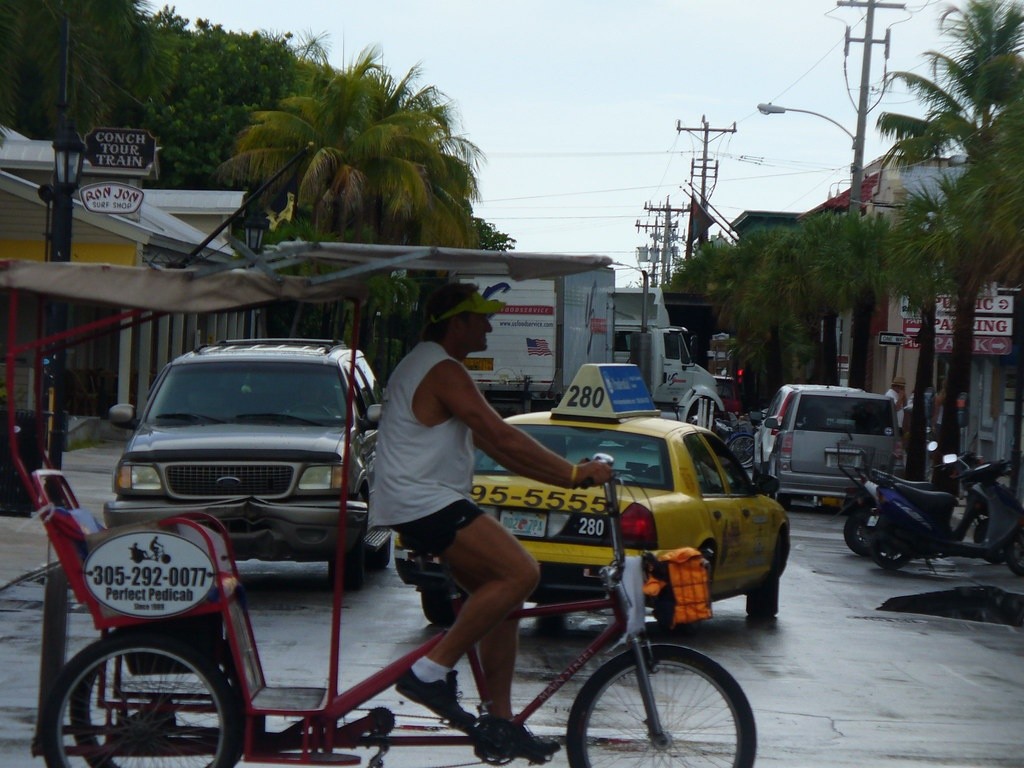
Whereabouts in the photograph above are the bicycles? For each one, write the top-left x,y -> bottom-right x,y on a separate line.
721,410 -> 773,471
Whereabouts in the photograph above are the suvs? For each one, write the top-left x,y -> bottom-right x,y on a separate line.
103,337 -> 393,594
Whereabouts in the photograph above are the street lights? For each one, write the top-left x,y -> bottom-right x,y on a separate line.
757,100 -> 866,391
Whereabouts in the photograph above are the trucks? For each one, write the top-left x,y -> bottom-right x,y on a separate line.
446,253 -> 719,434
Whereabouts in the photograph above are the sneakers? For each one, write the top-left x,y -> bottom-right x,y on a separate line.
476,715 -> 561,756
395,668 -> 477,728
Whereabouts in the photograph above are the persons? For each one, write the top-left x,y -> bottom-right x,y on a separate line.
366,282 -> 614,754
931,377 -> 948,438
884,376 -> 907,433
287,377 -> 338,416
715,366 -> 722,375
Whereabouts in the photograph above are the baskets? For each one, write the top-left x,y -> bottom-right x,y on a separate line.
645,555 -> 714,628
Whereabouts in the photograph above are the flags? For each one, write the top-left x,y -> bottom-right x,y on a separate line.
686,197 -> 716,259
233,168 -> 299,233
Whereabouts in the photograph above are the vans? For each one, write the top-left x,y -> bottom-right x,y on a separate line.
751,384 -> 906,516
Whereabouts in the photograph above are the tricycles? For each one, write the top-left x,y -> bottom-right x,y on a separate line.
0,243 -> 756,768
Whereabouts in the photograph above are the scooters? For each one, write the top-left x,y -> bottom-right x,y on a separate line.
838,444 -> 1024,579
831,432 -> 1008,573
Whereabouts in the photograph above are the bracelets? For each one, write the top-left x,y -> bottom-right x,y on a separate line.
570,464 -> 578,489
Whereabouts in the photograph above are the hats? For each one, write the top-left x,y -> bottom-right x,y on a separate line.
430,291 -> 507,324
892,377 -> 907,387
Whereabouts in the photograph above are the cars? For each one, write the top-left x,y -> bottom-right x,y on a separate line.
713,374 -> 742,421
390,362 -> 790,640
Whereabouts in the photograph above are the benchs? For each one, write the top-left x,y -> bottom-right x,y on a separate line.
34,469 -> 243,630
